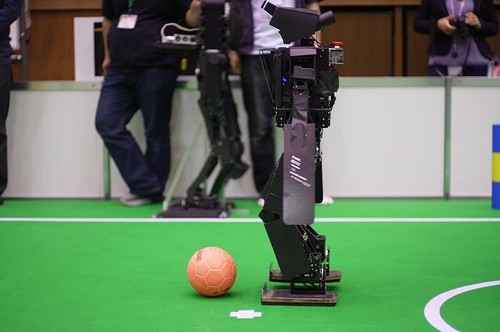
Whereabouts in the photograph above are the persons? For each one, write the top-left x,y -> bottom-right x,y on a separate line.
257,0 -> 343,308
94,0 -> 201,206
412,0 -> 500,76
0,0 -> 22,206
224,0 -> 334,207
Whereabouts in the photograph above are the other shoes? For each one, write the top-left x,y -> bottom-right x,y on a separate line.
121,195 -> 150,206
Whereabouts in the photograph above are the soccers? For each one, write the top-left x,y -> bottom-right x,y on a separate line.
186,247 -> 237,297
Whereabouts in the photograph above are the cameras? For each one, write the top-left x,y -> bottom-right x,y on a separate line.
449,16 -> 470,38
